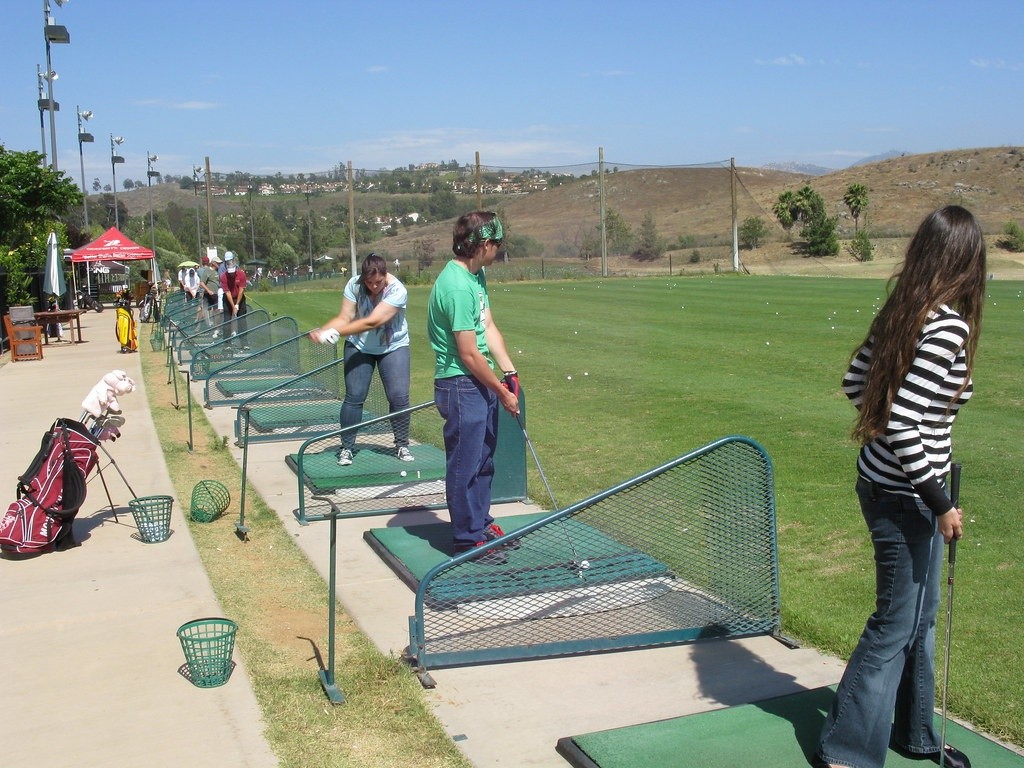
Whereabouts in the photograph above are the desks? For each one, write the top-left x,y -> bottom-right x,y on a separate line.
33,309 -> 87,347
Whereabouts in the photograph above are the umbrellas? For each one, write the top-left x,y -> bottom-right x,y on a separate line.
43,229 -> 67,340
177,260 -> 200,268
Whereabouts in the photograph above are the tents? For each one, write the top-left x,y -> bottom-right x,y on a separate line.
72,226 -> 159,310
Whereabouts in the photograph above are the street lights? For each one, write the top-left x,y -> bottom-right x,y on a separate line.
146,155 -> 160,253
38,69 -> 60,169
44,0 -> 72,173
304,187 -> 312,267
78,109 -> 95,238
110,136 -> 124,231
194,166 -> 203,265
247,177 -> 257,271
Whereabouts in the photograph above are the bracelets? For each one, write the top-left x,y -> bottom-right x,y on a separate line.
503,371 -> 517,376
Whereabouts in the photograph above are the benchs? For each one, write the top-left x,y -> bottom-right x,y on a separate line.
4,314 -> 44,361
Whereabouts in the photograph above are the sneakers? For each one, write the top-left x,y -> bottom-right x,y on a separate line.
483,523 -> 522,550
335,448 -> 353,466
454,538 -> 510,566
393,446 -> 414,462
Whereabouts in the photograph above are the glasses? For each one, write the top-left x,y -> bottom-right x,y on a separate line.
490,239 -> 502,246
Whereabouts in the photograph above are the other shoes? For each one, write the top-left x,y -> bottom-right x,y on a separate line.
811,752 -> 832,768
212,330 -> 224,339
928,742 -> 972,768
244,345 -> 250,350
224,346 -> 231,351
231,331 -> 237,338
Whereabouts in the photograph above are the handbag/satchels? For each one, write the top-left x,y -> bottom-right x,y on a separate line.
115,305 -> 139,352
138,295 -> 153,322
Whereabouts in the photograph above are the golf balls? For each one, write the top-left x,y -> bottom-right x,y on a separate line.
581,560 -> 589,568
401,471 -> 407,477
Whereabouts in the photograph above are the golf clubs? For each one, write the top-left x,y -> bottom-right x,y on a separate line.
245,294 -> 278,316
938,461 -> 972,768
164,313 -> 211,359
232,314 -> 237,337
89,405 -> 126,443
505,374 -> 580,567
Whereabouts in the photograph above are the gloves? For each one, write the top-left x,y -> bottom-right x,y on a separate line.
500,370 -> 519,401
235,304 -> 240,311
318,328 -> 340,346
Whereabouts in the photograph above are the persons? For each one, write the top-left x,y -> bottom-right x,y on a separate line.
308,253 -> 415,465
178,252 -> 251,351
426,211 -> 523,566
162,267 -> 172,294
812,205 -> 986,768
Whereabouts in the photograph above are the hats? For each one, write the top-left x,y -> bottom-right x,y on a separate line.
149,282 -> 155,287
224,251 -> 234,261
189,269 -> 194,277
202,256 -> 209,262
211,256 -> 223,264
227,260 -> 237,273
